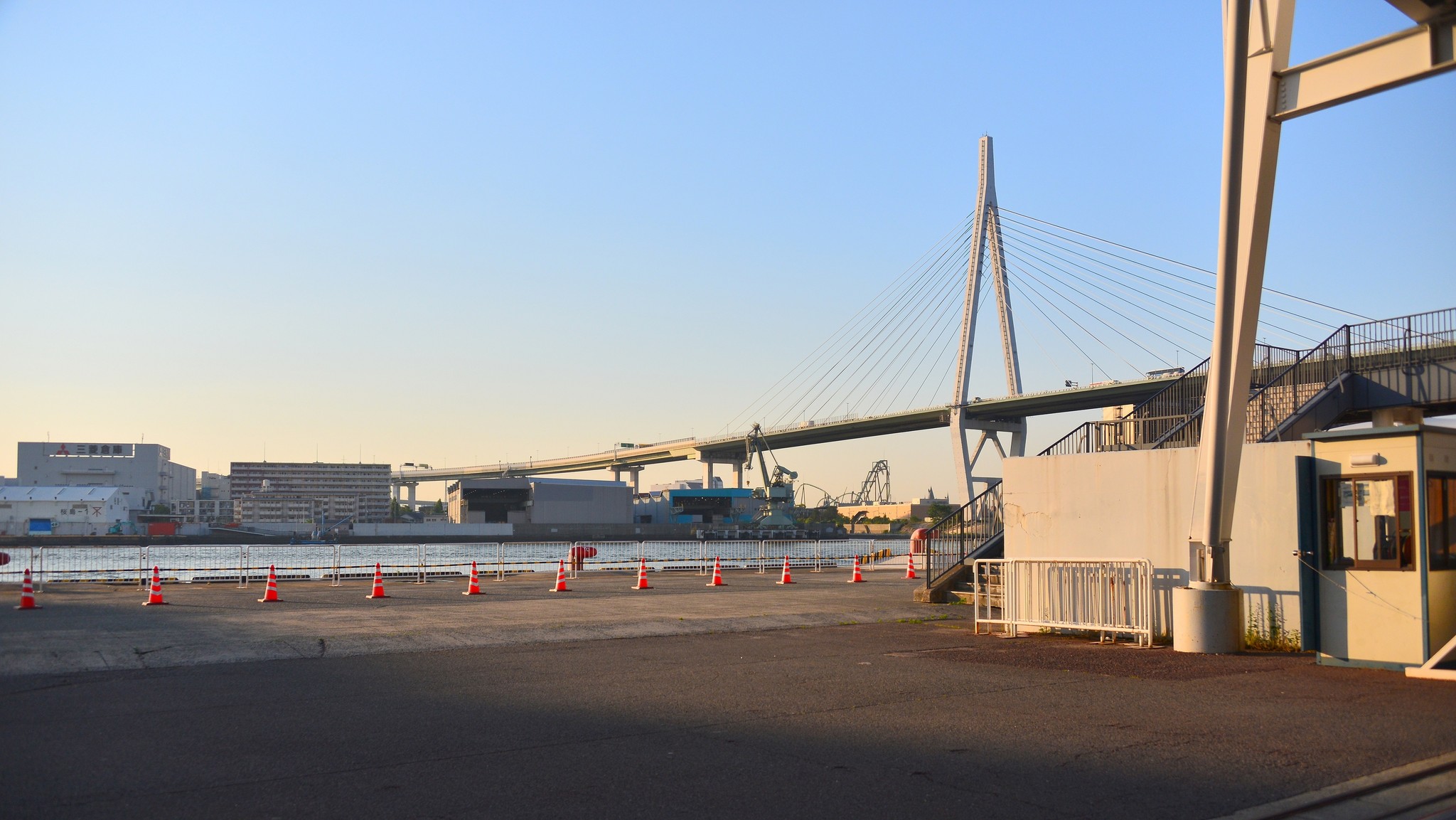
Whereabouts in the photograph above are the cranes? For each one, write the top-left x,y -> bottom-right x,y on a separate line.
743,423 -> 799,528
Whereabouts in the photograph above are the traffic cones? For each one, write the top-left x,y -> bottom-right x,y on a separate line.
706,556 -> 728,586
257,565 -> 283,602
365,563 -> 391,599
14,569 -> 43,610
776,555 -> 797,585
142,565 -> 170,606
462,561 -> 487,596
847,555 -> 867,583
901,553 -> 921,579
630,558 -> 654,589
549,559 -> 573,592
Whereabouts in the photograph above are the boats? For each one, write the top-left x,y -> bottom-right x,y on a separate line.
289,509 -> 354,544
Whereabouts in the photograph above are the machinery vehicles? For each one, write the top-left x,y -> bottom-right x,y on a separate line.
105,521 -> 138,535
